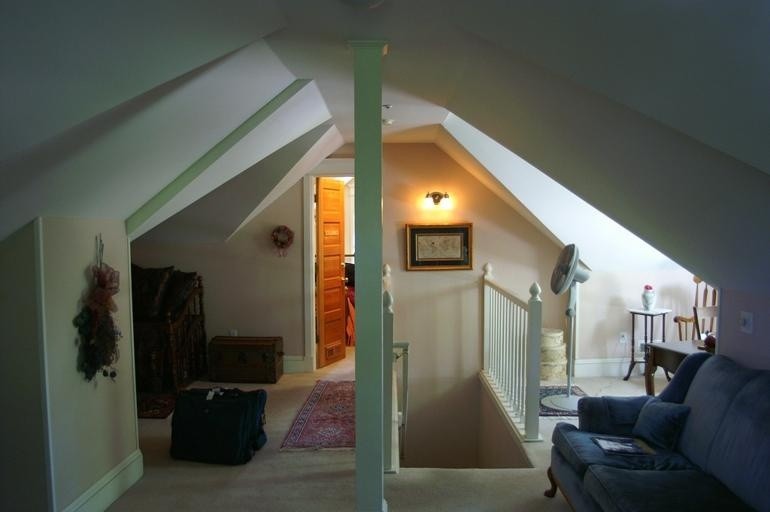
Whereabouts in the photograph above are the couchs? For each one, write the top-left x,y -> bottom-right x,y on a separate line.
543,352 -> 770,510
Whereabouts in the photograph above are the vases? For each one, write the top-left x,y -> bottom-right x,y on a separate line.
641,290 -> 657,312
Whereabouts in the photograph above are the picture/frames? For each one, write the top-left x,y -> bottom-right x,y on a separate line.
405,223 -> 474,271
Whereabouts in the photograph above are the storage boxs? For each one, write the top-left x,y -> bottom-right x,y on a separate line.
208,335 -> 284,384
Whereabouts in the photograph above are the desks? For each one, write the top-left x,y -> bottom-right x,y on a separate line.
644,339 -> 709,397
623,308 -> 672,382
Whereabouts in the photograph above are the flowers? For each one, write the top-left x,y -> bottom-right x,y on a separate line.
644,285 -> 653,290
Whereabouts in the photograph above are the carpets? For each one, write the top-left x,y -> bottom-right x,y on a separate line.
539,384 -> 590,417
279,379 -> 355,452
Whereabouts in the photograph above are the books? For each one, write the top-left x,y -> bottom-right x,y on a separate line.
591,435 -> 657,457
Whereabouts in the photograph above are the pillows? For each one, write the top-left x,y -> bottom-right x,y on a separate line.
632,397 -> 691,452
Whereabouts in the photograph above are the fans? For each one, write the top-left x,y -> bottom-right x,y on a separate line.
540,244 -> 593,412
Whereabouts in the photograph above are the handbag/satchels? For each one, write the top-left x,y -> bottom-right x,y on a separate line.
171,387 -> 267,465
